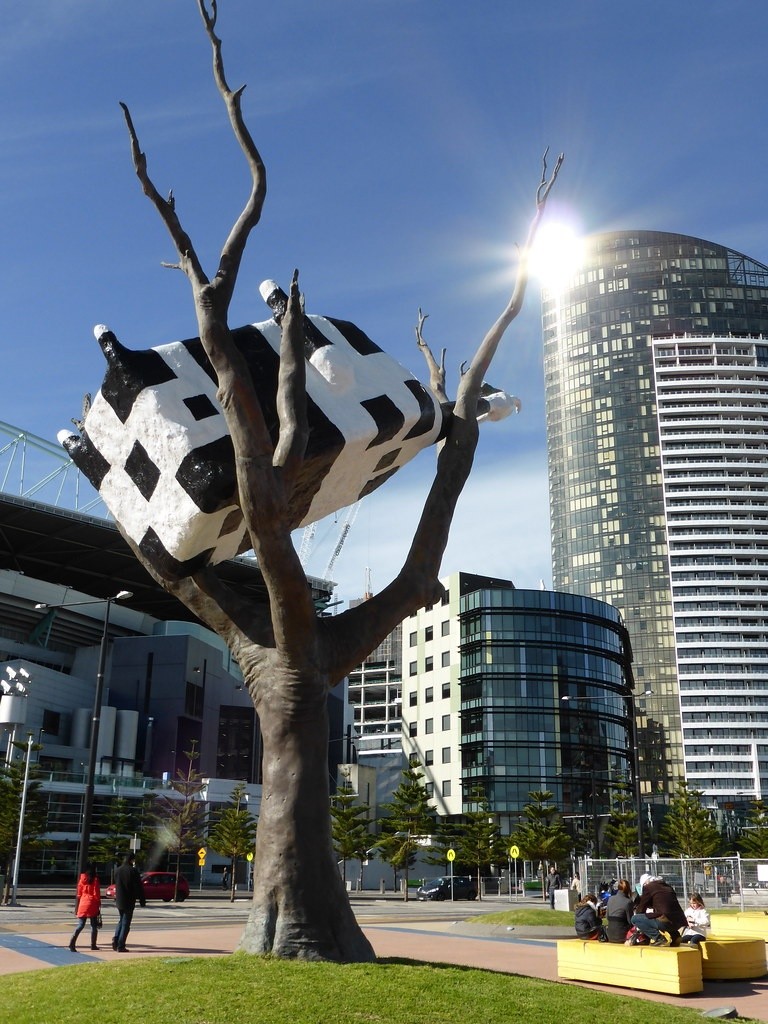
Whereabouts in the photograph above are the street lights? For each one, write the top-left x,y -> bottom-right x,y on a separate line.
34,590 -> 134,914
555,769 -> 616,903
561,691 -> 654,877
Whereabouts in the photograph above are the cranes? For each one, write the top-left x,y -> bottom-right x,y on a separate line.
299,498 -> 363,580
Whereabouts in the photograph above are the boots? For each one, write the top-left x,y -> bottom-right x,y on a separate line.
68,930 -> 81,952
91,931 -> 99,950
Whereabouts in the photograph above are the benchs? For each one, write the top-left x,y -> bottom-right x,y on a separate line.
557,913 -> 768,995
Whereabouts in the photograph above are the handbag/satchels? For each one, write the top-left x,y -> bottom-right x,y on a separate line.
628,930 -> 650,945
95,911 -> 102,929
597,925 -> 609,942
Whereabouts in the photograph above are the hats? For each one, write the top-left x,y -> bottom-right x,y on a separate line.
640,874 -> 651,886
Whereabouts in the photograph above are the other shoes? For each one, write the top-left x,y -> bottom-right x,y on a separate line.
112,937 -> 119,950
650,935 -> 668,946
117,947 -> 129,952
670,936 -> 682,947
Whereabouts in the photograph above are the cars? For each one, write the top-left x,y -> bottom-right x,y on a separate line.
105,871 -> 189,903
416,875 -> 478,902
661,876 -> 689,897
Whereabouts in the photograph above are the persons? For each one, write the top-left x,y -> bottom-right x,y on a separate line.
545,867 -> 562,909
112,852 -> 145,952
69,860 -> 101,952
250,867 -> 253,891
570,863 -> 733,947
222,867 -> 228,891
